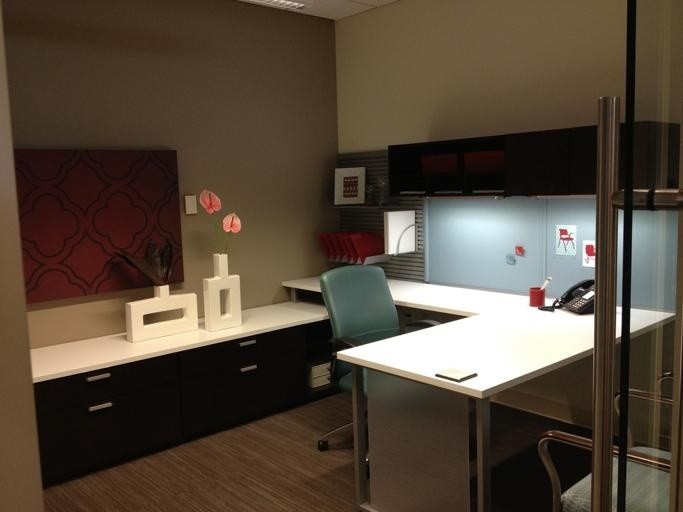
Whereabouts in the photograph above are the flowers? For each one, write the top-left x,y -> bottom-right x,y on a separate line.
197,188 -> 246,256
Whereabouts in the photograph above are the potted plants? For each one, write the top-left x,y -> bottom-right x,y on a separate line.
109,239 -> 198,344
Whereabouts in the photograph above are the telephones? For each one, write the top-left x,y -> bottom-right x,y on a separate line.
559,280 -> 596,313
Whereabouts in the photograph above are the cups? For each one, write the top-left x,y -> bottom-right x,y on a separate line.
529,287 -> 546,308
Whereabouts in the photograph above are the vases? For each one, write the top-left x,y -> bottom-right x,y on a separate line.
203,252 -> 242,331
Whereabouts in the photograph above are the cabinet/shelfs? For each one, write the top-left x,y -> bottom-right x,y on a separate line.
32,355 -> 183,489
178,332 -> 278,447
268,318 -> 335,417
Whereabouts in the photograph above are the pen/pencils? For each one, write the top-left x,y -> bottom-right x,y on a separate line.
540,277 -> 552,290
